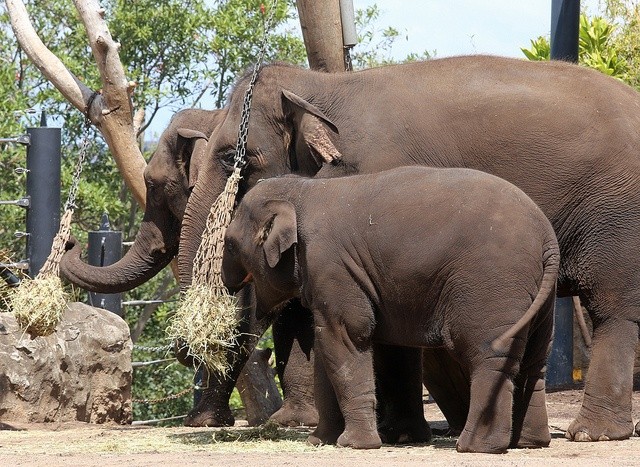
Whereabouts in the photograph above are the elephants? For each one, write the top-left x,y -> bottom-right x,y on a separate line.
56,105 -> 471,437
179,53 -> 640,443
220,163 -> 562,455
